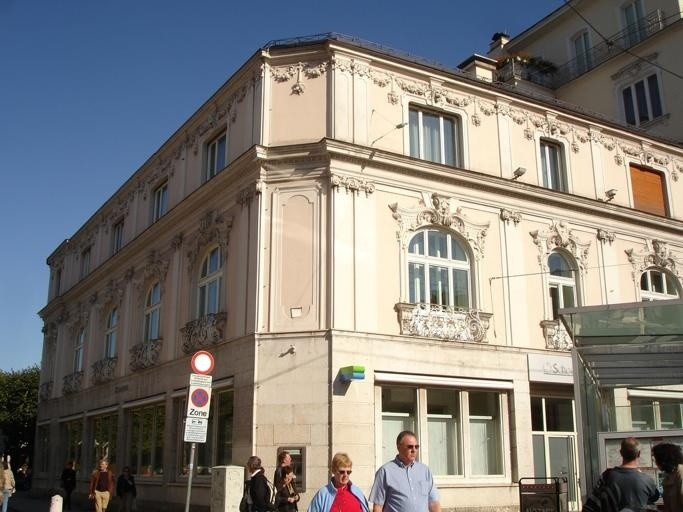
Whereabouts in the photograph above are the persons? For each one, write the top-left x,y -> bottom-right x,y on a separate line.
0,461 -> 32,512
368,430 -> 442,512
117,466 -> 137,512
592,437 -> 660,512
650,440 -> 683,512
273,449 -> 296,488
306,453 -> 370,512
252,467 -> 269,512
89,459 -> 116,512
60,461 -> 77,510
244,455 -> 275,512
276,466 -> 301,512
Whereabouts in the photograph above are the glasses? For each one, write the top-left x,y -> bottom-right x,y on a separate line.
335,468 -> 353,475
400,444 -> 420,449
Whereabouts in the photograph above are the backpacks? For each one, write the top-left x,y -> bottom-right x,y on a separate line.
265,479 -> 281,512
581,469 -> 626,512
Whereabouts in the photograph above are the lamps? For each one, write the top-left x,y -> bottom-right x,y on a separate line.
372,122 -> 408,145
604,188 -> 619,203
511,167 -> 526,180
